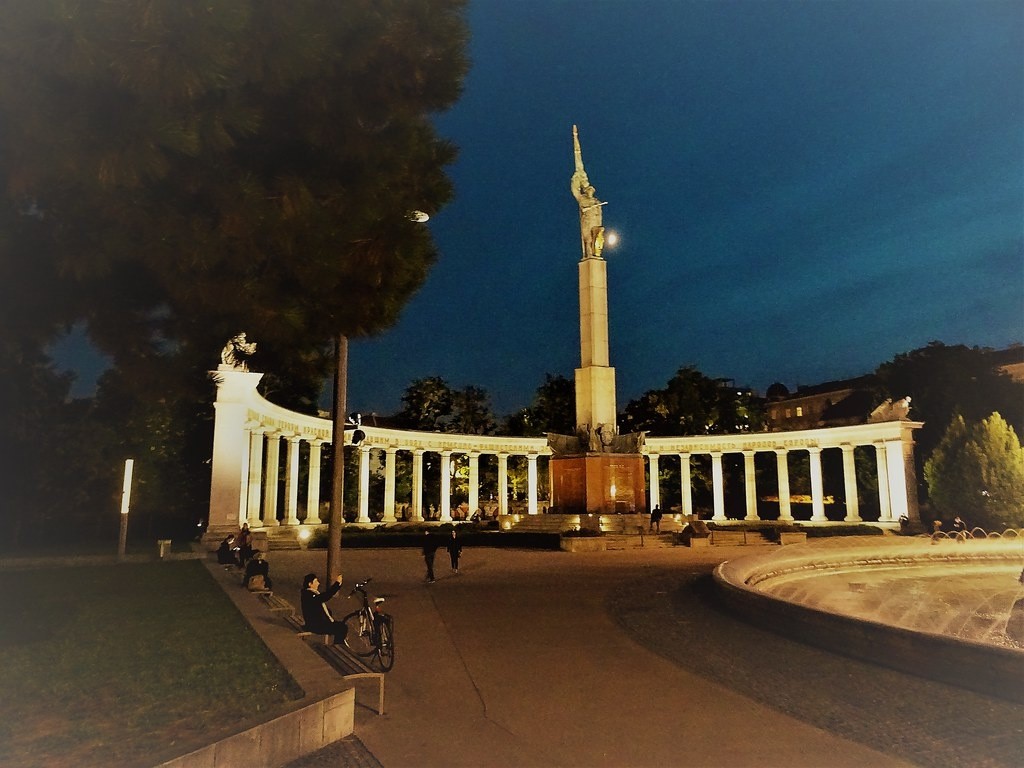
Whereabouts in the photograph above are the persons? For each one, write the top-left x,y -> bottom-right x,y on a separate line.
577,186 -> 603,259
447,529 -> 462,573
241,553 -> 274,591
216,535 -> 241,563
649,503 -> 662,534
239,523 -> 249,547
301,572 -> 348,644
954,517 -> 966,540
422,529 -> 436,584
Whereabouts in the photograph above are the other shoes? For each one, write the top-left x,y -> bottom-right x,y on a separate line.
452,569 -> 458,573
428,579 -> 435,583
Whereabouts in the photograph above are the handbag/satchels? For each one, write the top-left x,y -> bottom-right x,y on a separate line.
248,575 -> 265,591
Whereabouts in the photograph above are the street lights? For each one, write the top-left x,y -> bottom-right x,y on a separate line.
328,207 -> 430,598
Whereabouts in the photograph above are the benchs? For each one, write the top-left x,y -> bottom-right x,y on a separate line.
259,595 -> 296,616
223,564 -> 273,596
310,644 -> 384,716
282,614 -> 329,645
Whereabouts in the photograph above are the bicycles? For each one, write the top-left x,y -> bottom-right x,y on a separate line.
341,577 -> 395,674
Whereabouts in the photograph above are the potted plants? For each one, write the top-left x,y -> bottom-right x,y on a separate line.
560,527 -> 607,553
775,524 -> 807,546
